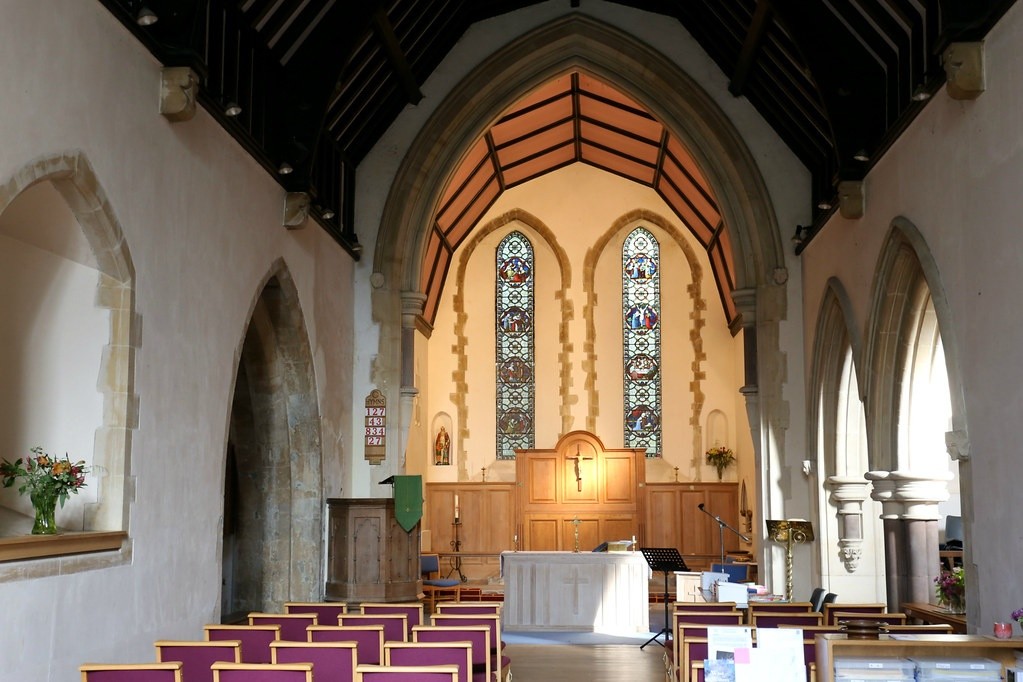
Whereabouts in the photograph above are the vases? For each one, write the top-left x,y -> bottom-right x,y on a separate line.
717,463 -> 723,482
944,595 -> 966,614
30,493 -> 58,534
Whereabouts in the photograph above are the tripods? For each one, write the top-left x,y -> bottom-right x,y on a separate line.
639,547 -> 692,649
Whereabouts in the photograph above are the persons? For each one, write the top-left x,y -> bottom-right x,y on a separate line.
435,427 -> 450,465
567,453 -> 592,479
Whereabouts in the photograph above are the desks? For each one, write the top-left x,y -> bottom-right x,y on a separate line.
815,634 -> 1023,682
499,550 -> 652,633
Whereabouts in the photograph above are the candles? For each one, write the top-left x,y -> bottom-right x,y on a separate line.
454,495 -> 458,518
632,535 -> 635,542
514,535 -> 517,542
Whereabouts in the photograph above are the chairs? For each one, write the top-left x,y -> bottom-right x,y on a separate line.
663,588 -> 954,682
78,601 -> 511,682
421,554 -> 460,616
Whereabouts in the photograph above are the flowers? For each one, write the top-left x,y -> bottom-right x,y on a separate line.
0,448 -> 91,527
1009,608 -> 1023,623
933,569 -> 966,610
706,447 -> 736,478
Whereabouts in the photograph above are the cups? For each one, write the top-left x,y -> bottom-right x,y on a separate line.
994,623 -> 1012,639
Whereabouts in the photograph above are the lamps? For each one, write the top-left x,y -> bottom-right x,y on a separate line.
771,69 -> 935,244
136,5 -> 364,250
